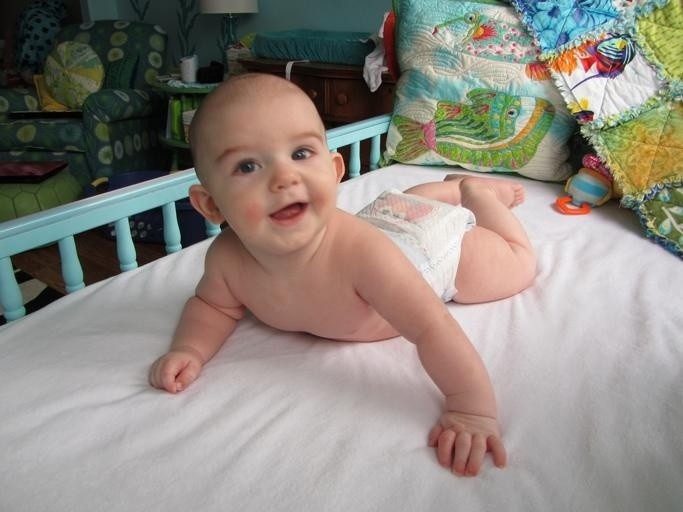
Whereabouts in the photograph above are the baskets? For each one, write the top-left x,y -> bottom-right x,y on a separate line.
85,169 -> 204,245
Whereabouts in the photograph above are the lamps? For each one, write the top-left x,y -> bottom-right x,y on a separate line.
198,0 -> 259,45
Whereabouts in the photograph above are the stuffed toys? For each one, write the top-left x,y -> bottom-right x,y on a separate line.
556,154 -> 620,216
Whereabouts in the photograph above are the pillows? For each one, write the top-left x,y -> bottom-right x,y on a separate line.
374,0 -> 578,186
34,37 -> 142,114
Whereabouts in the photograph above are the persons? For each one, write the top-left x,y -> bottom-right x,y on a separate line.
148,74 -> 540,480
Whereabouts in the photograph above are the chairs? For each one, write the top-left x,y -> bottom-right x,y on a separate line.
0,18 -> 169,189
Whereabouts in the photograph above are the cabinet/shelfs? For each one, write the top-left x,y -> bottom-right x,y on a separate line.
236,56 -> 396,184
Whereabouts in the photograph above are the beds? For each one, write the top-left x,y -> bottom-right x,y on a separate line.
1,113 -> 683,512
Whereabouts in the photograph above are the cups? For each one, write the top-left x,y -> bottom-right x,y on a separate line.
179,54 -> 199,84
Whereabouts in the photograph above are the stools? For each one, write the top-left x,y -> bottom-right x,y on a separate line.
0,171 -> 82,250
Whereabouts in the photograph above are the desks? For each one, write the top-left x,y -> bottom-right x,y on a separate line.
151,83 -> 221,172
